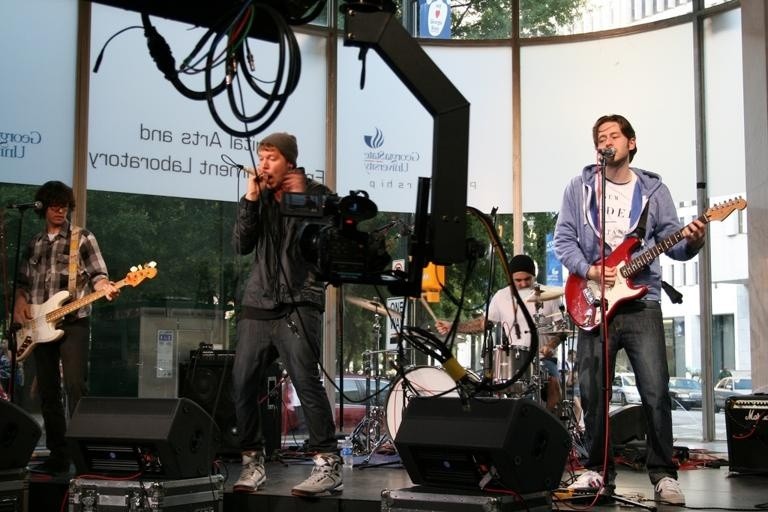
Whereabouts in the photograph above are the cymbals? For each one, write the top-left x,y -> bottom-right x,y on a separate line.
521,287 -> 564,302
540,326 -> 580,337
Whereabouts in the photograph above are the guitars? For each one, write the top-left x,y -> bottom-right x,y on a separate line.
7,260 -> 158,362
564,196 -> 746,331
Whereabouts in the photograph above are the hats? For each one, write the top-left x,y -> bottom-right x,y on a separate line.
259,131 -> 298,168
507,254 -> 536,277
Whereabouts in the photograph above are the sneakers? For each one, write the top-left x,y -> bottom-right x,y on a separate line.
653,476 -> 686,505
567,469 -> 616,492
290,451 -> 345,497
232,450 -> 267,493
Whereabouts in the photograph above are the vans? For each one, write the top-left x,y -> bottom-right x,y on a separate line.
281,377 -> 391,434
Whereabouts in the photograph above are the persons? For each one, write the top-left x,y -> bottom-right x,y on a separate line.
0,337 -> 14,403
553,115 -> 705,506
232,132 -> 345,498
11,180 -> 121,476
436,255 -> 569,414
559,349 -> 576,400
718,367 -> 732,388
567,361 -> 582,425
29,358 -> 64,400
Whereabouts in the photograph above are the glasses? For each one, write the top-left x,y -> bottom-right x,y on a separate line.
47,203 -> 73,213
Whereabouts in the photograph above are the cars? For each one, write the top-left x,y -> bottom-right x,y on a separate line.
713,377 -> 756,411
608,371 -> 643,406
666,377 -> 703,409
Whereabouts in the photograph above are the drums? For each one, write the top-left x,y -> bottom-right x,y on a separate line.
387,364 -> 465,443
482,344 -> 540,394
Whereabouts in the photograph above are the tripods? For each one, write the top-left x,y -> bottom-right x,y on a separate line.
347,319 -> 586,469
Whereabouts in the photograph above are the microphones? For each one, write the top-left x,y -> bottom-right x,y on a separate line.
598,147 -> 616,159
241,166 -> 269,182
6,201 -> 43,210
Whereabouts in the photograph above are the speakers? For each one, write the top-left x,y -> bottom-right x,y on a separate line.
608,403 -> 647,444
189,349 -> 281,460
725,395 -> 768,478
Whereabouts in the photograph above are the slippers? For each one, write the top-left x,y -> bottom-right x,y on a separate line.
25,462 -> 70,475
